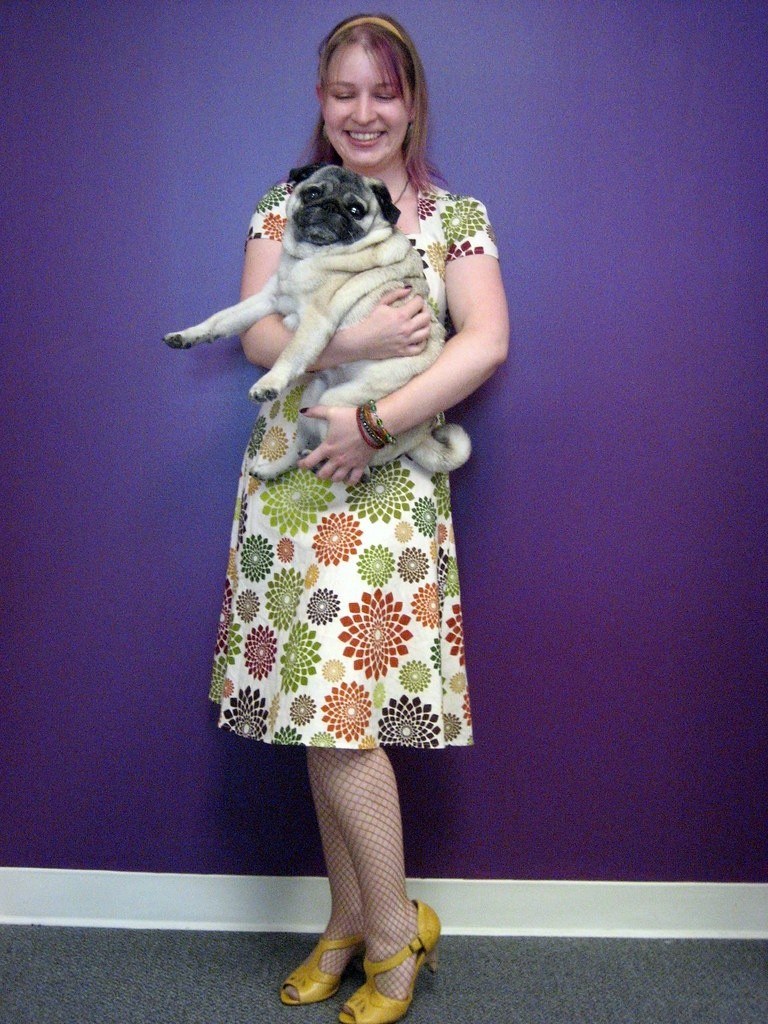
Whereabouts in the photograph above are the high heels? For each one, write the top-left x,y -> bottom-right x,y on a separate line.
338,898 -> 441,1024
279,933 -> 365,1005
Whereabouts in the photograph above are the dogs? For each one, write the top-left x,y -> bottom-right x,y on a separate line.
159,162 -> 474,486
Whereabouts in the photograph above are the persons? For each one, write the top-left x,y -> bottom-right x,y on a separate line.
207,12 -> 510,1024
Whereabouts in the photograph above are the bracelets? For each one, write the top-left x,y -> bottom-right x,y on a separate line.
356,400 -> 398,450
